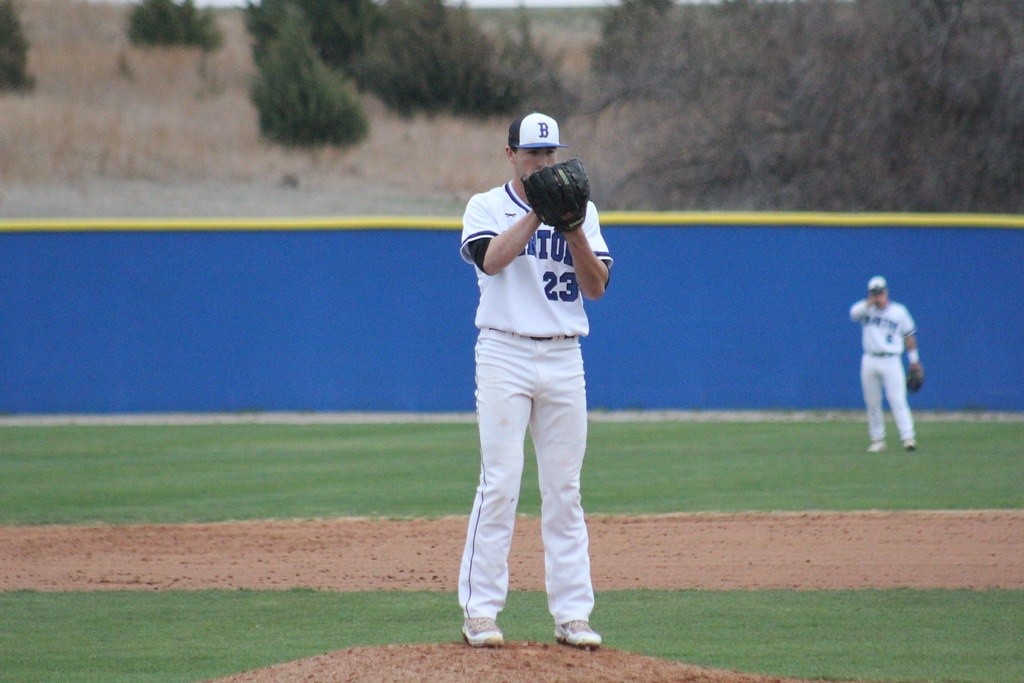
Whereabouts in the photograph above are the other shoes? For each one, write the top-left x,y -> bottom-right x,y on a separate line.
866,440 -> 887,454
903,438 -> 917,451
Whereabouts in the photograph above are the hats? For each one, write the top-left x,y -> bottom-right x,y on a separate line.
867,275 -> 887,292
508,111 -> 568,147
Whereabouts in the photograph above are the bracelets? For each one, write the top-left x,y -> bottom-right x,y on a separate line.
907,351 -> 919,365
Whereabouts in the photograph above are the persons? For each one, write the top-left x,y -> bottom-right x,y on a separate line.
457,112 -> 613,646
848,276 -> 923,453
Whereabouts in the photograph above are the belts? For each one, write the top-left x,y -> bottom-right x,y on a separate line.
869,353 -> 895,357
488,329 -> 576,342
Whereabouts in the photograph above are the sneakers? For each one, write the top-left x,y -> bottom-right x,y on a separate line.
462,617 -> 504,647
554,620 -> 602,648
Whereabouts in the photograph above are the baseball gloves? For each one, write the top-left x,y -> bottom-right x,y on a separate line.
905,367 -> 924,392
523,157 -> 593,232
